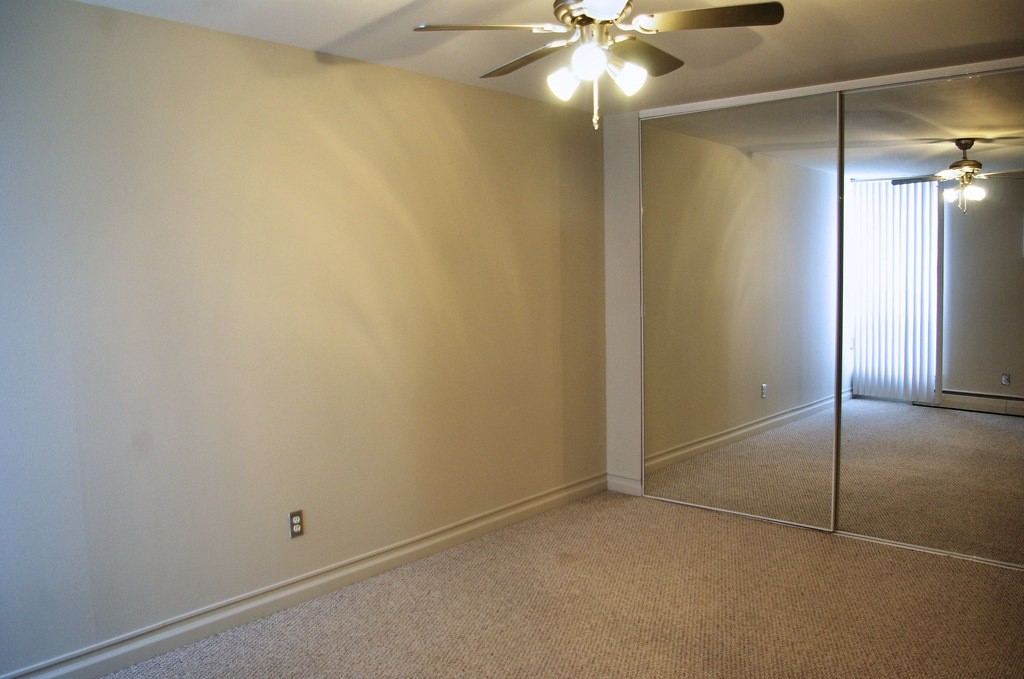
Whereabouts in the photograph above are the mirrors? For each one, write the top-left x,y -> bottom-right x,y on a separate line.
833,50 -> 1024,571
640,80 -> 847,542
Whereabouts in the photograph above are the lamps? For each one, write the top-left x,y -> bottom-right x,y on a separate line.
571,41 -> 607,81
546,66 -> 580,101
942,175 -> 986,203
607,57 -> 646,96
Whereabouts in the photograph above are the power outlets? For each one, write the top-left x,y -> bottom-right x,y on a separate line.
761,383 -> 767,398
1000,372 -> 1010,386
289,509 -> 303,539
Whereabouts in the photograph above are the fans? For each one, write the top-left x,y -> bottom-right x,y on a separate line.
890,137 -> 1024,185
411,0 -> 784,80
